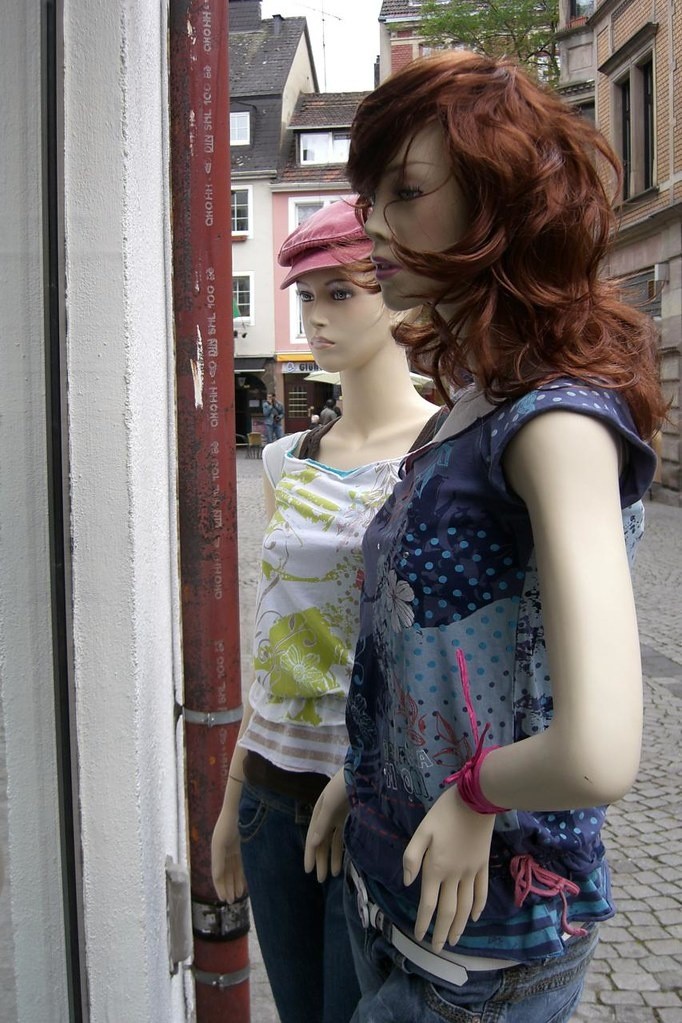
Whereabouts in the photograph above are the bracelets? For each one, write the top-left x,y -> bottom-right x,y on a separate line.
445,647 -> 509,814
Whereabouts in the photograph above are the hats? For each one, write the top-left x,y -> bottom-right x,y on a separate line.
276,195 -> 372,290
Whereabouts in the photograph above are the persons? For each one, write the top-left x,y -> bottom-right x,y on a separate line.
212,195 -> 442,1022
303,57 -> 664,1022
309,399 -> 342,429
262,392 -> 285,444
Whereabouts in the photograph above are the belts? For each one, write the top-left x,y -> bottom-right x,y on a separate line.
348,860 -> 588,987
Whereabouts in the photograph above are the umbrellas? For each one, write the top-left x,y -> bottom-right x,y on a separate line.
304,369 -> 433,386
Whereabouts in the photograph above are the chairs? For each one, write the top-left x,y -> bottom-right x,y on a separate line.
247,432 -> 266,458
